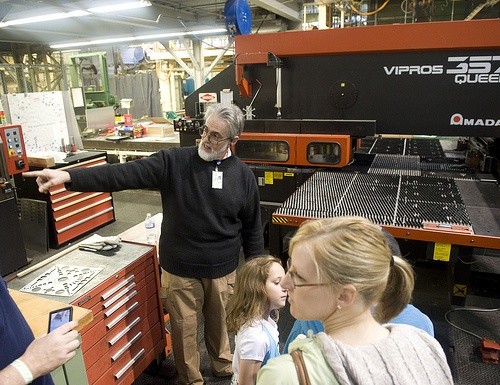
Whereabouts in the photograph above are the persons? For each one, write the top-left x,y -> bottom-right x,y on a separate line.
0,273 -> 80,385
224,255 -> 289,385
256,216 -> 454,385
22,102 -> 265,385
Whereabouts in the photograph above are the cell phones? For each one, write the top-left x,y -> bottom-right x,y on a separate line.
48,307 -> 73,334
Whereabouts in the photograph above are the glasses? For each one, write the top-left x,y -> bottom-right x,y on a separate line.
286,259 -> 330,289
199,125 -> 233,145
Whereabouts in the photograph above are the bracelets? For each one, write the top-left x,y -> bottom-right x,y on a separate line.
10,359 -> 33,384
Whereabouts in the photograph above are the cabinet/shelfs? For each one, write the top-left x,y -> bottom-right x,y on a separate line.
5,237 -> 166,385
13,152 -> 116,249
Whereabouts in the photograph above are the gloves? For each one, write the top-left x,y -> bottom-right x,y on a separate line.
79,236 -> 121,252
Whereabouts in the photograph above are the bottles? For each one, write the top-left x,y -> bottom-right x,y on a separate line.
124,127 -> 133,140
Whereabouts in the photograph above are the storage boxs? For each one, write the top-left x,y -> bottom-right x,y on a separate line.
146,117 -> 174,139
